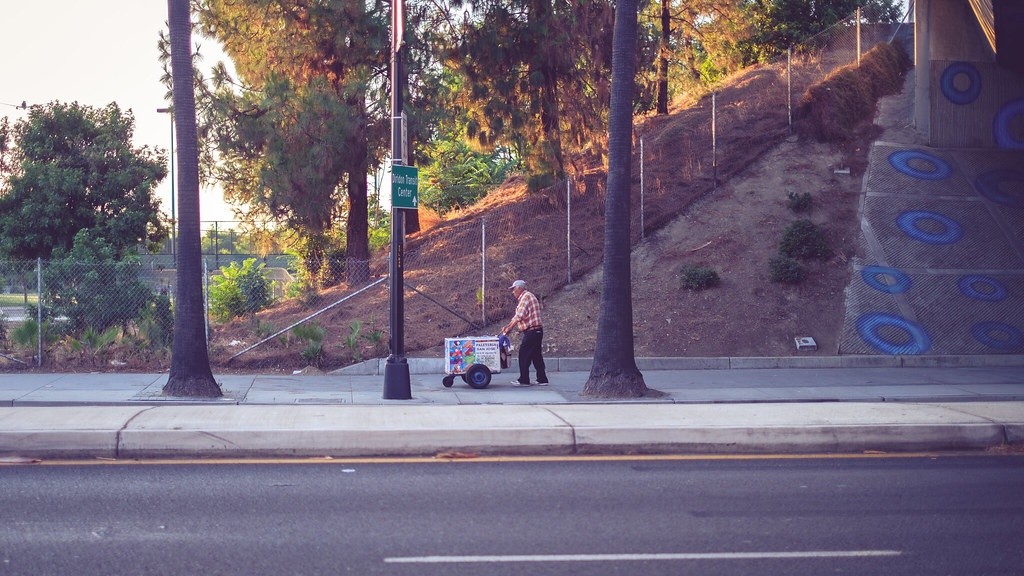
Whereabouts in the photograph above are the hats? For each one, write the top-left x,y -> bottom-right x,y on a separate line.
508,280 -> 526,289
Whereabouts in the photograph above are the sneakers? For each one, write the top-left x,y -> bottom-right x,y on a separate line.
534,381 -> 549,386
511,380 -> 530,387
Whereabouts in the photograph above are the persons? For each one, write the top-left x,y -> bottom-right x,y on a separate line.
502,280 -> 549,387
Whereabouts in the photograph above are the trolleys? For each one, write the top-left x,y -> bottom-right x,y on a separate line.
442,330 -> 501,389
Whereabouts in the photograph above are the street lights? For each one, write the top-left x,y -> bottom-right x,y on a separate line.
157,107 -> 175,254
